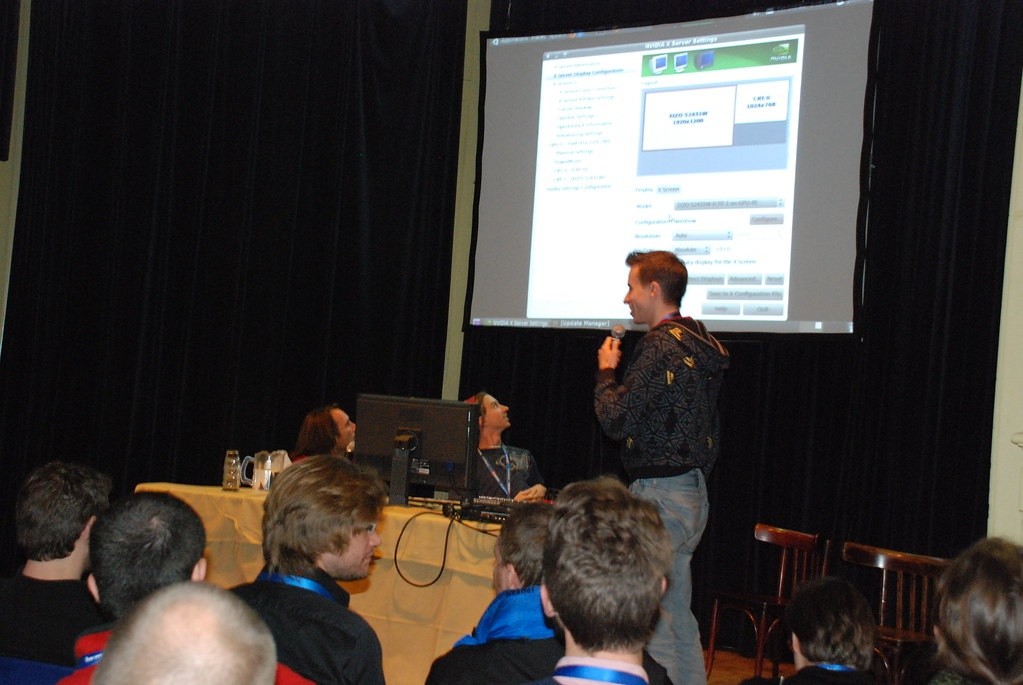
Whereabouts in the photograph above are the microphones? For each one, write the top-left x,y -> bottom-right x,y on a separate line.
610,324 -> 625,349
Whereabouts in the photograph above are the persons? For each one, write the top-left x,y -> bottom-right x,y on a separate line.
0,460 -> 106,669
927,540 -> 1023,685
54,491 -> 316,685
742,576 -> 877,685
465,392 -> 547,501
520,476 -> 667,685
290,403 -> 356,463
228,455 -> 386,685
90,581 -> 277,685
594,250 -> 729,685
424,501 -> 674,685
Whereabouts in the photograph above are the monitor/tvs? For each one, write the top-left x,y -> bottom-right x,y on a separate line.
352,391 -> 480,509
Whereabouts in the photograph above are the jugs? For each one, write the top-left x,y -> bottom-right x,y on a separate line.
241,450 -> 288,491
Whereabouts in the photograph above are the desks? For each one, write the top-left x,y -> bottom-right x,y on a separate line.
134,481 -> 498,685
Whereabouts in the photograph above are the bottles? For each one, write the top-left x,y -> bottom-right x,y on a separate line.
221,450 -> 241,490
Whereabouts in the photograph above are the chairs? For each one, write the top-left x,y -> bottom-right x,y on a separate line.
705,523 -> 832,681
842,542 -> 951,685
